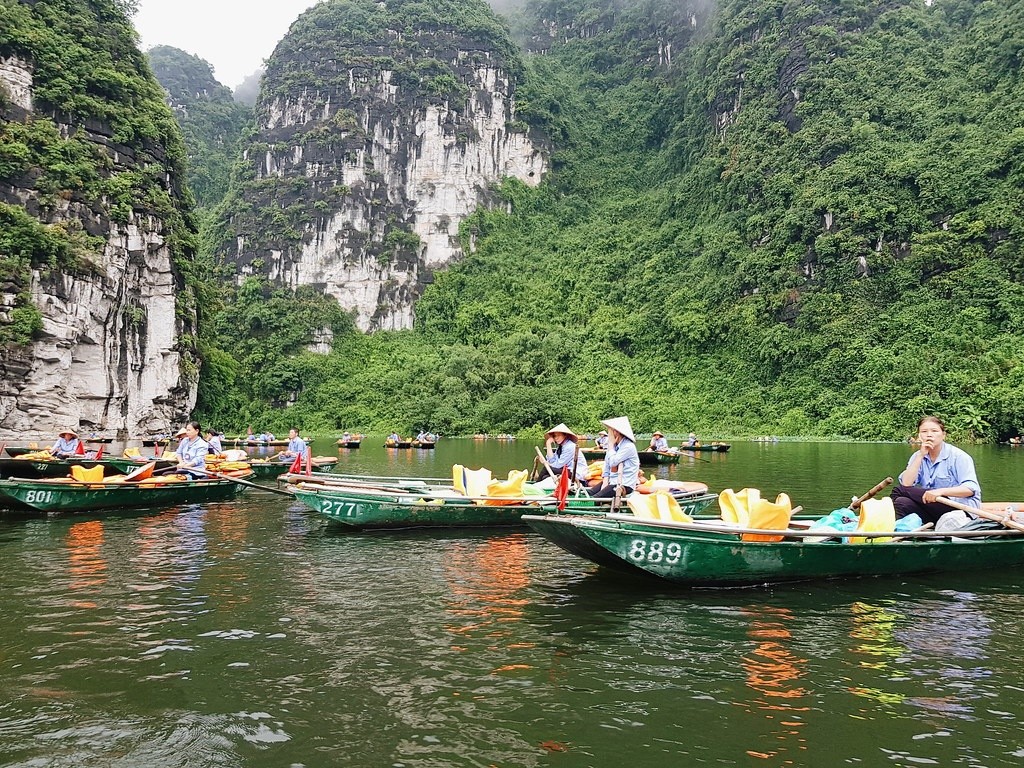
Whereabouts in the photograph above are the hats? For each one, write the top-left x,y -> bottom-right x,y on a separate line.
598,430 -> 608,435
544,423 -> 578,443
176,427 -> 187,436
59,428 -> 79,439
653,431 -> 664,438
343,431 -> 349,434
599,415 -> 636,442
209,428 -> 223,435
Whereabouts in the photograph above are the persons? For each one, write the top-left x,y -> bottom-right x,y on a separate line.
162,421 -> 208,481
498,433 -> 513,438
889,415 -> 981,529
536,423 -> 588,496
247,424 -> 275,441
218,431 -> 225,441
175,427 -> 188,442
153,432 -> 166,441
586,416 -> 640,506
594,430 -> 609,449
417,431 -> 434,442
647,431 -> 668,452
689,433 -> 698,447
49,429 -> 78,460
758,436 -> 778,441
388,431 -> 398,442
343,432 -> 351,441
278,427 -> 307,462
205,428 -> 222,455
480,433 -> 488,438
90,433 -> 96,439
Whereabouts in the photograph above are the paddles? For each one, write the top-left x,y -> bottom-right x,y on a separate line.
152,466 -> 177,475
264,451 -> 285,461
293,452 -> 325,471
569,443 -> 580,487
665,448 -> 711,464
183,467 -> 294,496
935,495 -> 1024,533
535,445 -> 559,486
613,460 -> 624,512
205,437 -> 224,455
846,476 -> 894,511
530,456 -> 538,480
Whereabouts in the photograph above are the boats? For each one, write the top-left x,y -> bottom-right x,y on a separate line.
1009,437 -> 1023,444
338,439 -> 361,449
541,445 -> 682,466
518,499 -> 1023,586
275,469 -> 724,532
413,441 -> 437,450
680,441 -> 734,452
243,457 -> 341,477
0,437 -> 315,513
385,440 -> 412,449
